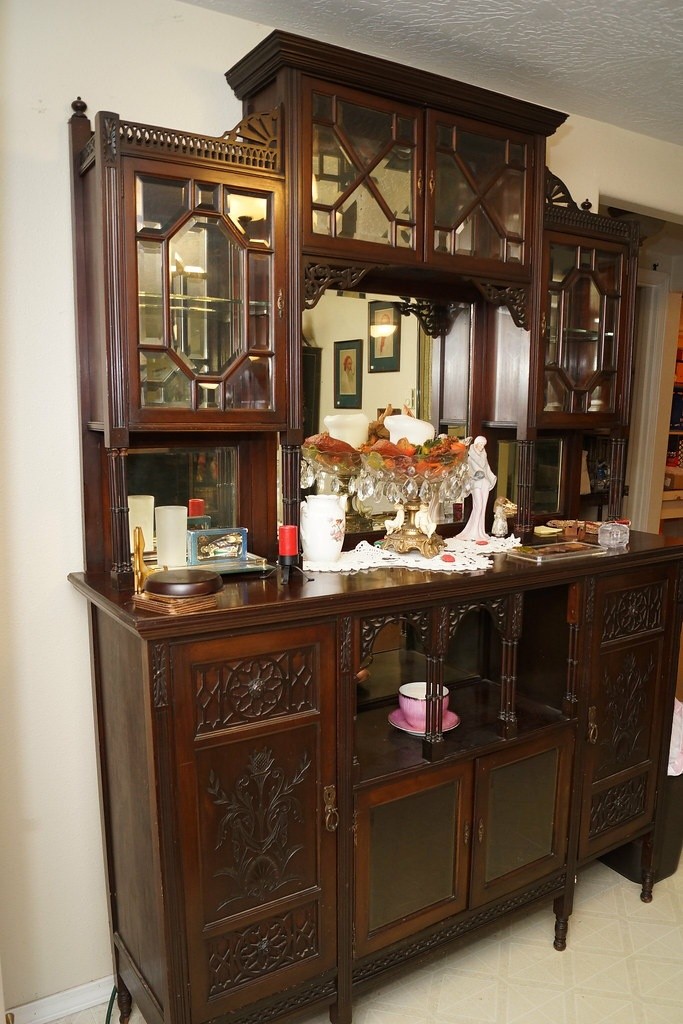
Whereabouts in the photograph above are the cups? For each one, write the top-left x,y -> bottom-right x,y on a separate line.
127,495 -> 154,554
155,506 -> 188,566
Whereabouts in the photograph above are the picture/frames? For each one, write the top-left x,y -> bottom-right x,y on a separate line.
366,301 -> 401,374
333,339 -> 362,410
188,529 -> 248,567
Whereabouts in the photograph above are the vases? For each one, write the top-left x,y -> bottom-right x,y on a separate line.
299,494 -> 349,563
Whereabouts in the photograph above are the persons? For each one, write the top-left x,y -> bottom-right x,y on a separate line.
457,436 -> 496,537
373,308 -> 395,359
338,354 -> 358,397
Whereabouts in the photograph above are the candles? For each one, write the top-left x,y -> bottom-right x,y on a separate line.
280,525 -> 298,557
189,499 -> 205,516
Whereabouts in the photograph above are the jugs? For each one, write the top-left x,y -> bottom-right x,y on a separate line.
300,495 -> 349,563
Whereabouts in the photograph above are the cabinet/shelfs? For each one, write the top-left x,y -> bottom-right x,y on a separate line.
66,30 -> 682,1022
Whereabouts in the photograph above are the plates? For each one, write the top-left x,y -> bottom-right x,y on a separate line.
388,708 -> 461,737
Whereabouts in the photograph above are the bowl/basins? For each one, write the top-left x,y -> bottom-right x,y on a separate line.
398,681 -> 450,729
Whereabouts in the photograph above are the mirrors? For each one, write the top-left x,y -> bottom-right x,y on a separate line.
126,446 -> 240,562
302,287 -> 474,535
